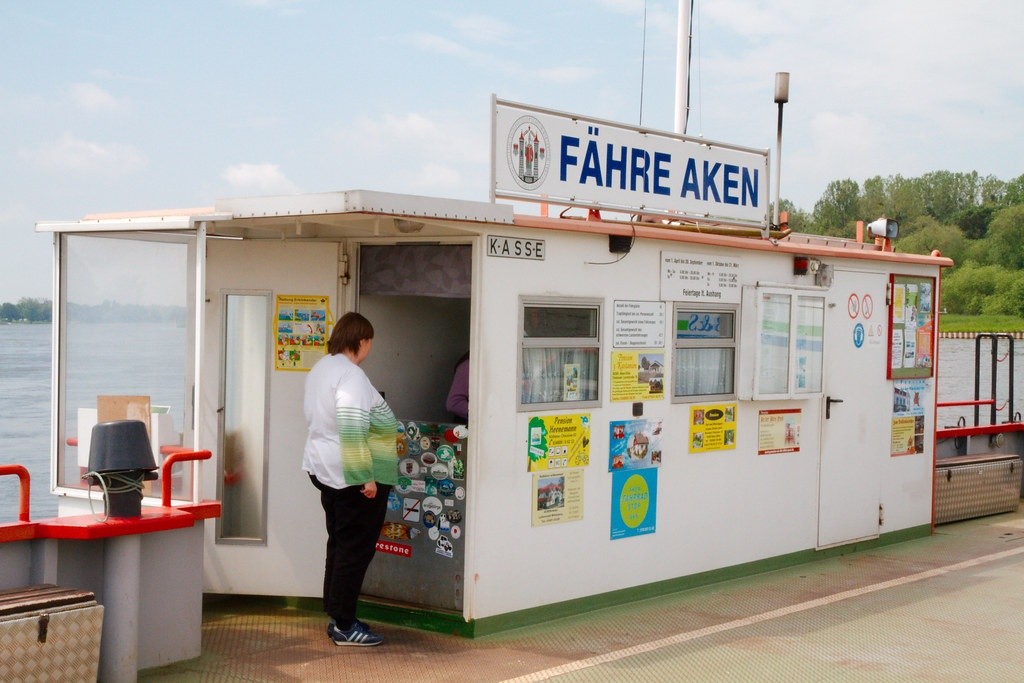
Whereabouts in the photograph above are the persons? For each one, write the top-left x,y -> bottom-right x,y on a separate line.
446,351 -> 469,425
302,313 -> 398,647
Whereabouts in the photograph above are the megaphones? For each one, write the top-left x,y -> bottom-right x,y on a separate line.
867,219 -> 898,238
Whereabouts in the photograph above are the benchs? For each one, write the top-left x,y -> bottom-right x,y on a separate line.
0,580 -> 106,683
935,451 -> 1023,522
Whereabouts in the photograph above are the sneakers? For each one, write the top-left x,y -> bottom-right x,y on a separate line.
331,624 -> 384,646
327,622 -> 370,638
